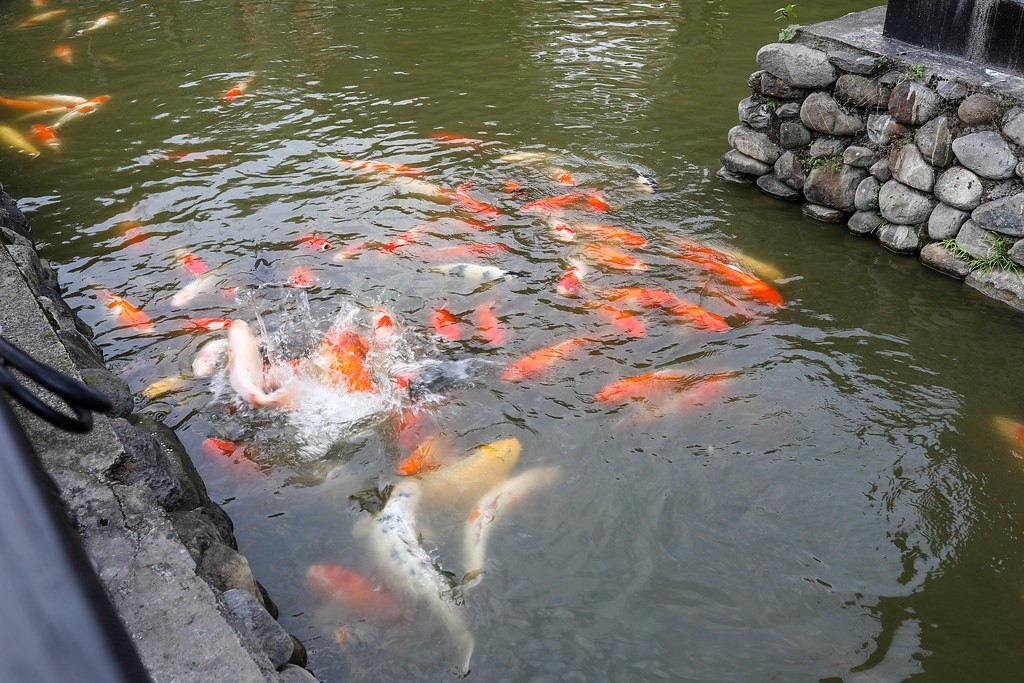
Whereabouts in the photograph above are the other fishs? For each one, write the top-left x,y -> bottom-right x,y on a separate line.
3,1 -> 788,668
992,416 -> 1024,456
